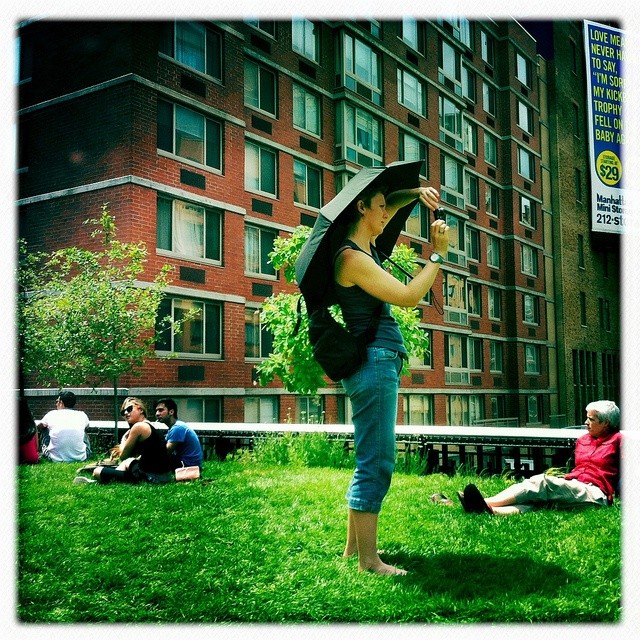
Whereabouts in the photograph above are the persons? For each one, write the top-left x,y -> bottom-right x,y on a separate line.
457,399 -> 623,516
154,398 -> 203,472
330,185 -> 450,578
40,391 -> 91,463
73,397 -> 171,486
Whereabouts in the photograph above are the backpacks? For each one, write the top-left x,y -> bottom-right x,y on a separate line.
292,238 -> 385,383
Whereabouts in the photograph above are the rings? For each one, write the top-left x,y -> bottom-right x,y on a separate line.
439,231 -> 444,233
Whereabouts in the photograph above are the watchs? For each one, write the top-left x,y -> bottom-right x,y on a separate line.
428,252 -> 443,265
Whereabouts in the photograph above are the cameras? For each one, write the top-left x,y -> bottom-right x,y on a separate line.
433,208 -> 446,224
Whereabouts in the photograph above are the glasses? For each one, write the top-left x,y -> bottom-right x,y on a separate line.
120,405 -> 133,417
154,408 -> 164,414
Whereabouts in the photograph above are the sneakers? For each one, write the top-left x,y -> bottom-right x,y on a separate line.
464,484 -> 495,516
457,491 -> 468,513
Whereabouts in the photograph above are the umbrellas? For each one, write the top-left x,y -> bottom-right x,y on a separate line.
294,159 -> 445,325
